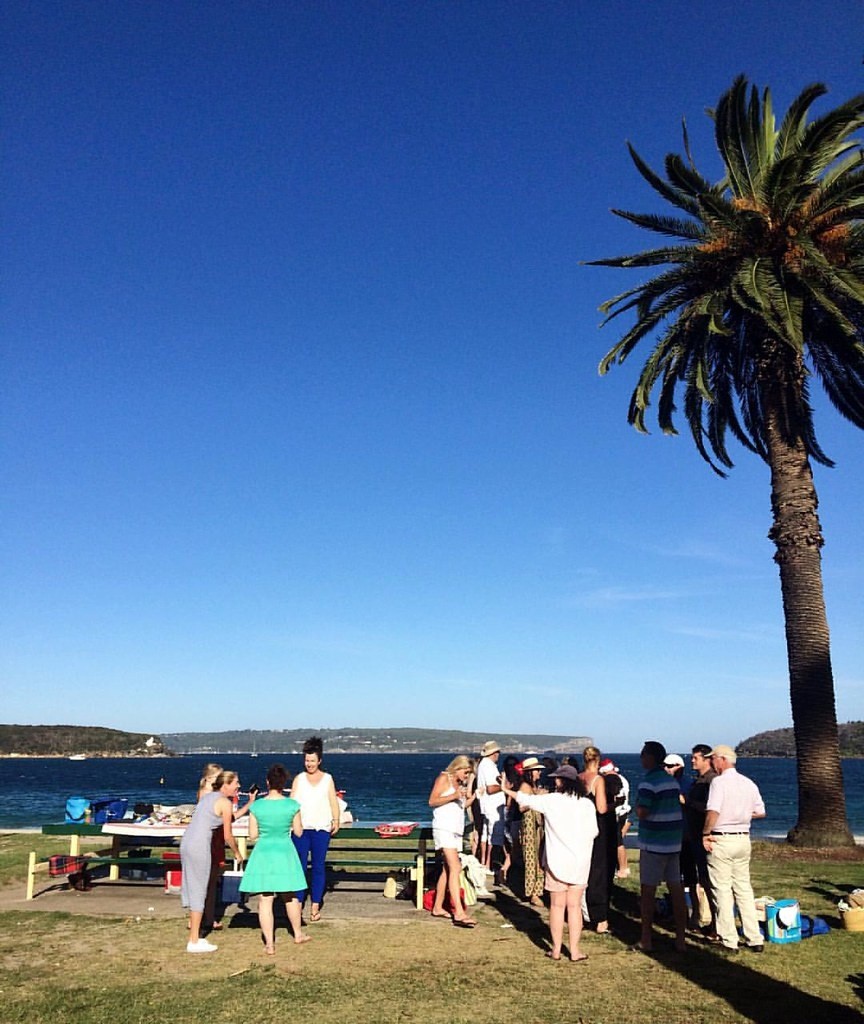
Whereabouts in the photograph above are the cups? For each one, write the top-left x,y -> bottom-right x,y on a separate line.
460,787 -> 468,800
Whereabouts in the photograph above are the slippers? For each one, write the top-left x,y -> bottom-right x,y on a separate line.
674,939 -> 688,956
431,909 -> 454,919
310,910 -> 320,921
453,917 -> 478,926
597,926 -> 617,934
545,950 -> 561,960
627,942 -> 653,950
571,953 -> 588,961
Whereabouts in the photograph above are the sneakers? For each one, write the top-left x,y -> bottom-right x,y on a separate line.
186,938 -> 219,953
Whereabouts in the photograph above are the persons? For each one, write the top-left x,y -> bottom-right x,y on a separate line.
429,755 -> 486,924
289,736 -> 340,922
498,764 -> 599,961
703,745 -> 766,955
186,763 -> 259,931
463,740 -> 721,942
179,770 -> 243,952
635,742 -> 687,950
240,765 -> 312,955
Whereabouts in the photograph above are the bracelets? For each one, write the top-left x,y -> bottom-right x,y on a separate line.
332,817 -> 339,820
703,833 -> 711,836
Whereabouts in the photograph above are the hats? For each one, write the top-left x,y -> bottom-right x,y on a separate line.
547,765 -> 577,780
704,745 -> 737,764
519,757 -> 546,770
480,741 -> 500,757
663,754 -> 685,767
599,759 -> 620,774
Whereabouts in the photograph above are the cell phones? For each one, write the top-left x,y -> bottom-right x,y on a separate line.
250,783 -> 261,795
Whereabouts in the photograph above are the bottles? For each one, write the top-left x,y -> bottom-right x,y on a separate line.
85,810 -> 91,823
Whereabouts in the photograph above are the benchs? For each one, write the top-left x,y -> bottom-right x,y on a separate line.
148,836 -> 254,849
328,843 -> 435,852
75,856 -> 184,866
319,856 -> 438,868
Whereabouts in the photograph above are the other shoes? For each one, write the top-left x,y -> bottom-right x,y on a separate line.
744,941 -> 763,952
187,919 -> 223,930
294,933 -> 313,943
266,944 -> 275,955
718,942 -> 739,954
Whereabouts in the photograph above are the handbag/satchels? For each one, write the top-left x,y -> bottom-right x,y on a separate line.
91,798 -> 128,824
423,888 -> 466,912
133,803 -> 153,820
384,866 -> 411,901
765,899 -> 802,944
65,797 -> 92,824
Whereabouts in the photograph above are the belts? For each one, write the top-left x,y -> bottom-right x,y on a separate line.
711,831 -> 750,835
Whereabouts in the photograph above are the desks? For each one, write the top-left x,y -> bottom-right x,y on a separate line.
26,816 -> 437,913
